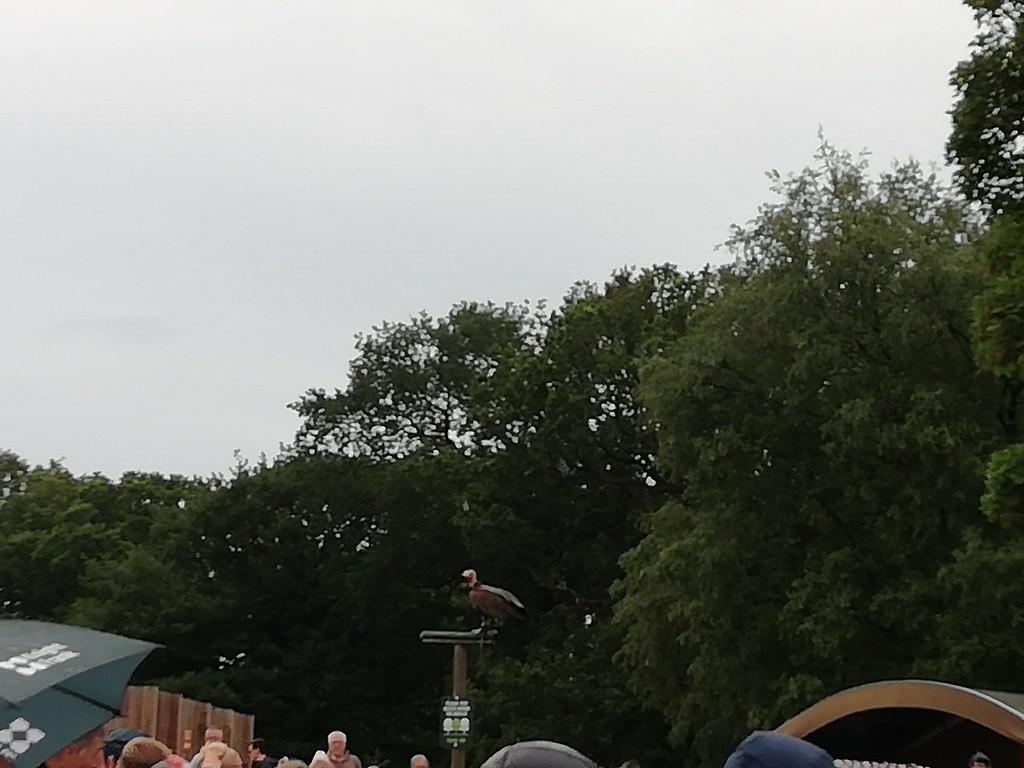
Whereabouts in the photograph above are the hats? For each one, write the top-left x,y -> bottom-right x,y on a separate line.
481,741 -> 597,767
103,728 -> 151,762
724,731 -> 834,768
117,737 -> 172,768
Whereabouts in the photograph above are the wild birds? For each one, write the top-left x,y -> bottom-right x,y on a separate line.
459,570 -> 528,636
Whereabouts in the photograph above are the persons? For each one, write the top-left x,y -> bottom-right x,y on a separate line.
969,751 -> 992,768
725,730 -> 837,768
411,754 -> 430,768
481,741 -> 604,768
103,725 -> 363,768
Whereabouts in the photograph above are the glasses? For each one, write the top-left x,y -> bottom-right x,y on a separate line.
247,749 -> 256,754
